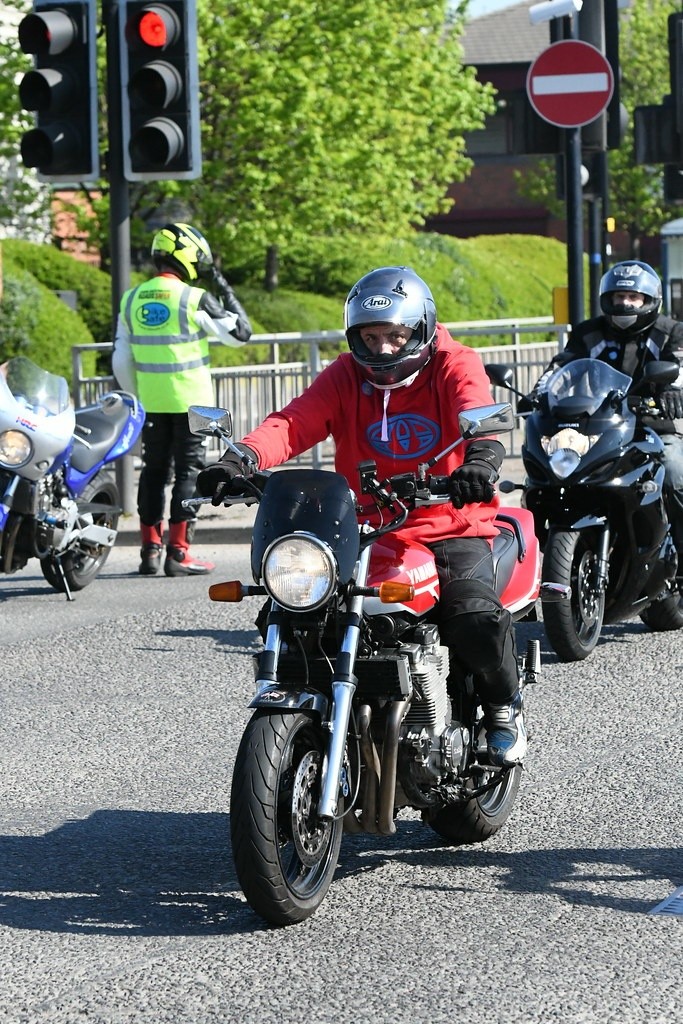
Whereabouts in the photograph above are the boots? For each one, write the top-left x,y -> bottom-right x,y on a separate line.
164,516 -> 218,578
139,517 -> 164,575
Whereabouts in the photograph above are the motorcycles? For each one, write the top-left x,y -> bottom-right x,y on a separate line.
484,357 -> 683,662
0,353 -> 148,604
176,400 -> 575,926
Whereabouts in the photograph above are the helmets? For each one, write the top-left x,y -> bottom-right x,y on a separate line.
599,262 -> 664,331
147,222 -> 215,284
347,270 -> 440,387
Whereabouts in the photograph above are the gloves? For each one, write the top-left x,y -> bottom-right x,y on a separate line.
654,385 -> 683,421
515,389 -> 540,421
204,254 -> 236,296
448,438 -> 508,508
199,443 -> 259,506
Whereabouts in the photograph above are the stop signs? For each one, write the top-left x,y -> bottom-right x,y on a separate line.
525,39 -> 614,130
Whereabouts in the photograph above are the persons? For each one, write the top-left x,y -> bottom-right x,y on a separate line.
198,267 -> 546,769
112,222 -> 251,577
534,261 -> 683,606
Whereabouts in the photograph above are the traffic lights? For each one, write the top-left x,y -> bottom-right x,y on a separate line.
17,0 -> 101,183
118,1 -> 203,182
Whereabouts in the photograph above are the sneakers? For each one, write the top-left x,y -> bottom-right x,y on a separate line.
485,686 -> 531,767
